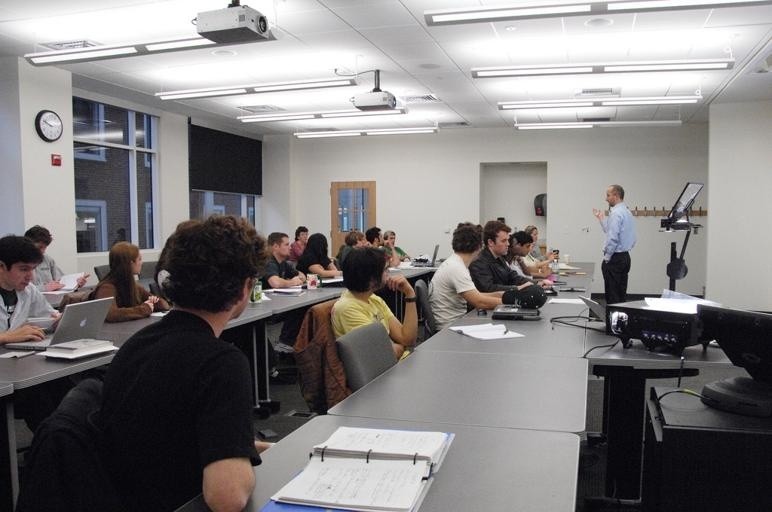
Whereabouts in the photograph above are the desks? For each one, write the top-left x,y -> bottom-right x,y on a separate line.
249,261 -> 453,409
327,352 -> 588,433
588,321 -> 771,509
464,261 -> 595,317
0,291 -> 272,511
415,318 -> 587,352
182,415 -> 581,511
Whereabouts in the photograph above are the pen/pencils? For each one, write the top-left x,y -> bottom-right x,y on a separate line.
504,327 -> 508,334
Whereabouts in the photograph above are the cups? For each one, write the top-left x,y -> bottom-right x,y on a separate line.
563,255 -> 570,264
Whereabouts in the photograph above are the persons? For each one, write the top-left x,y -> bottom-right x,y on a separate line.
23,225 -> 90,294
91,240 -> 170,322
592,184 -> 636,303
104,214 -> 276,512
0,235 -> 63,344
154,219 -> 201,305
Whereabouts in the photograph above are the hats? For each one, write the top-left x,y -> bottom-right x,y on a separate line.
500,284 -> 548,310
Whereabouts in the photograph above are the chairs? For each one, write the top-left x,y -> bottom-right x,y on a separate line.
94,261 -> 156,291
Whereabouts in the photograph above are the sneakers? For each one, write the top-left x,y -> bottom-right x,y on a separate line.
274,342 -> 295,355
270,367 -> 280,378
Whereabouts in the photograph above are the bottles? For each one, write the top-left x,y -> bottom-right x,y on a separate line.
306,273 -> 322,289
251,281 -> 262,302
551,259 -> 559,282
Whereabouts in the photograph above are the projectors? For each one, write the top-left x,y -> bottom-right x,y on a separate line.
193,2 -> 271,45
349,90 -> 398,110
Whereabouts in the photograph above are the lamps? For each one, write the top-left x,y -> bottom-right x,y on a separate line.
422,0 -> 772,28
152,77 -> 360,103
22,28 -> 280,69
514,120 -> 682,128
468,58 -> 735,80
294,126 -> 441,139
494,95 -> 704,110
234,105 -> 409,123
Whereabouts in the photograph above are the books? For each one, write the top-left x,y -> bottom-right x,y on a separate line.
41,272 -> 90,307
259,425 -> 456,512
37,336 -> 114,361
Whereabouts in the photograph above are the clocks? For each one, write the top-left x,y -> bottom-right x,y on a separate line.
36,109 -> 65,142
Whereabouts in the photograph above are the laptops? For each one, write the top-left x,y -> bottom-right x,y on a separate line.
411,243 -> 440,267
4,295 -> 115,352
492,304 -> 541,322
